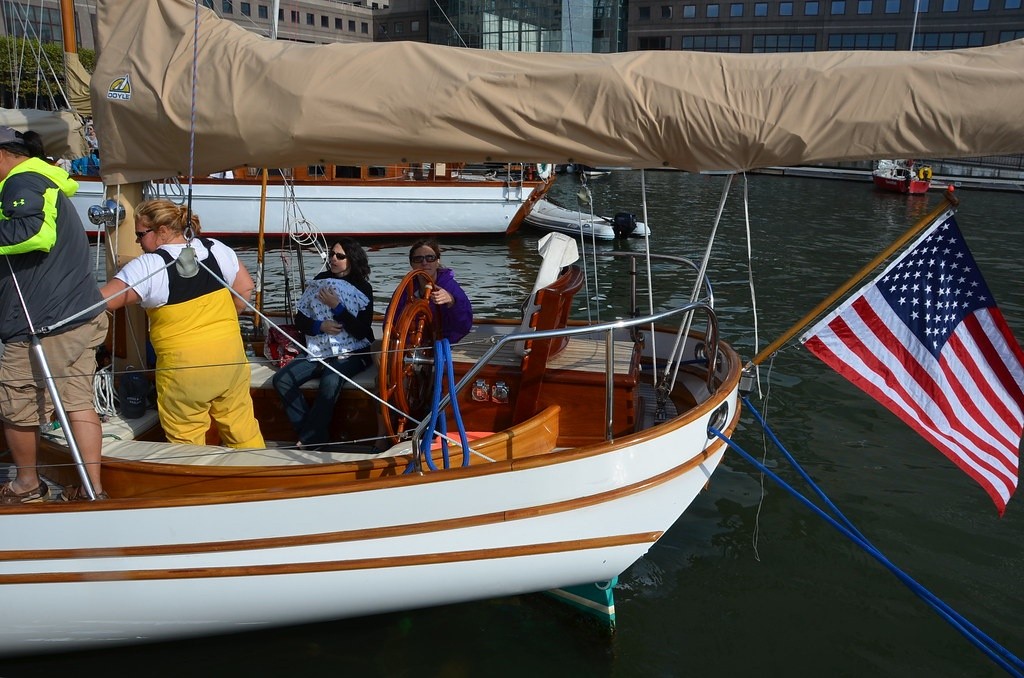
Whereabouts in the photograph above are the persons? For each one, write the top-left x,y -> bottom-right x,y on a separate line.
0,125 -> 110,504
273,237 -> 372,450
100,200 -> 266,449
366,241 -> 473,453
54,115 -> 100,176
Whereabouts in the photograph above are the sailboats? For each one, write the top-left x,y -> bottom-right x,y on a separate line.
0,0 -> 1024,666
0,1 -> 554,239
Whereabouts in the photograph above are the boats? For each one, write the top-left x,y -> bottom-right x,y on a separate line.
524,198 -> 651,243
872,159 -> 934,195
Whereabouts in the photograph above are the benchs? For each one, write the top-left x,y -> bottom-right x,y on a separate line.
36,336 -> 638,498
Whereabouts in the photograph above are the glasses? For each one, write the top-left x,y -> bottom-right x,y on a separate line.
329,252 -> 347,260
135,229 -> 153,239
412,255 -> 437,263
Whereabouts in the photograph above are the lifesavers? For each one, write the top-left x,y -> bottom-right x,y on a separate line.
536,163 -> 553,180
919,167 -> 932,182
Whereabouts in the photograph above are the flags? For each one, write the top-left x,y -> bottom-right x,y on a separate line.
799,207 -> 1024,519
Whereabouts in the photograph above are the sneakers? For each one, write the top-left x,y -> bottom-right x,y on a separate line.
61,485 -> 111,501
0,480 -> 51,503
